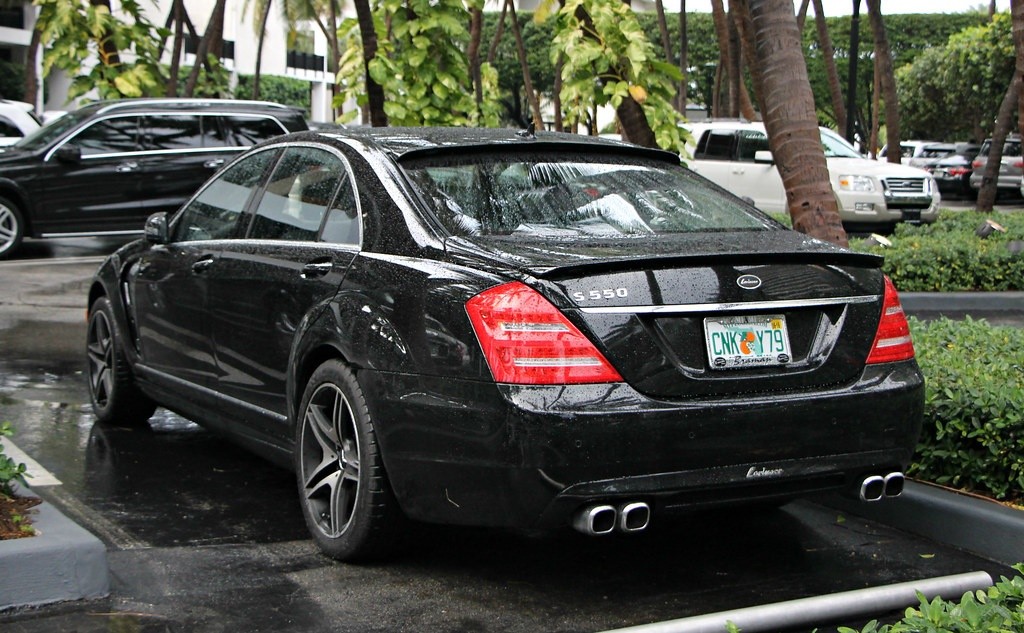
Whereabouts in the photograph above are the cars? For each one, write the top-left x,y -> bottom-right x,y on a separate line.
0,100 -> 48,153
878,133 -> 1024,203
669,120 -> 941,236
83,124 -> 926,563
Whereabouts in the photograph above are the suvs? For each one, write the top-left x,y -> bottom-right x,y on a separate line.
0,97 -> 317,259
